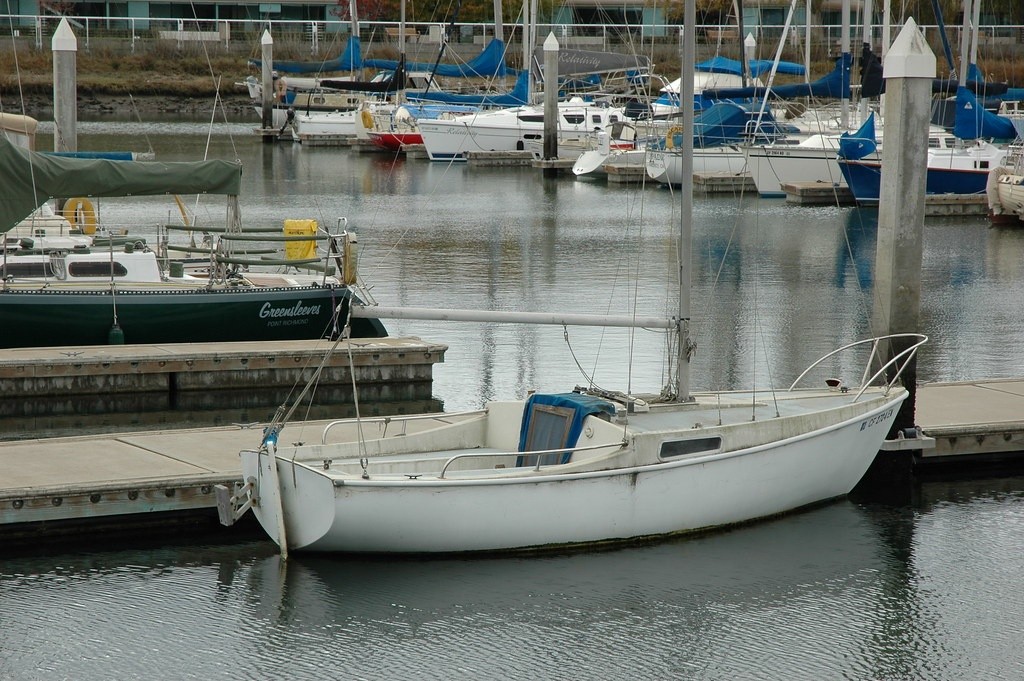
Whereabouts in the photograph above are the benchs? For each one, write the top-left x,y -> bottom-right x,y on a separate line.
383,27 -> 422,45
706,30 -> 738,44
959,30 -> 989,45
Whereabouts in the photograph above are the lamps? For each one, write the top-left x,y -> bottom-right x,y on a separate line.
823,378 -> 842,390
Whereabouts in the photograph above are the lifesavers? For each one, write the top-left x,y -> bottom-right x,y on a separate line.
665,126 -> 684,148
362,110 -> 374,129
62,197 -> 97,233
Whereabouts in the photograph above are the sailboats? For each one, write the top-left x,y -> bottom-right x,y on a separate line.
0,0 -> 1024,348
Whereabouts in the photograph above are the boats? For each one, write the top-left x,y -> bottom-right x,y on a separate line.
212,330 -> 931,566
